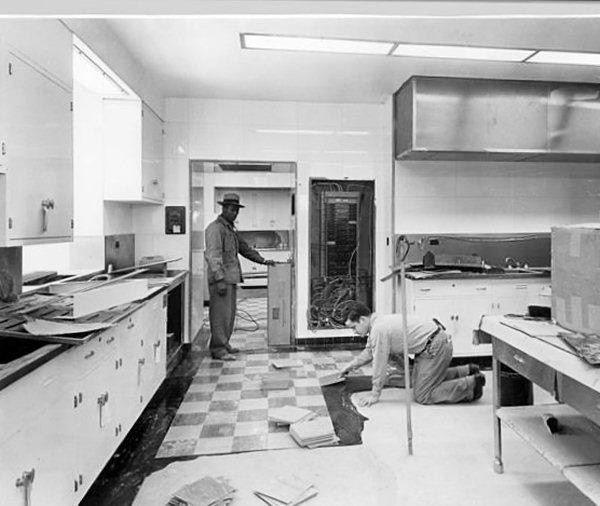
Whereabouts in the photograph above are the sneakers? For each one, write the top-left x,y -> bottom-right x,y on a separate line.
229,347 -> 240,353
212,351 -> 236,361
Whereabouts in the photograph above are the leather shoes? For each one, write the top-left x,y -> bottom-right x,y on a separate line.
469,364 -> 486,400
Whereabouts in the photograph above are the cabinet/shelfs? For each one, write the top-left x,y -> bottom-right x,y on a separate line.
216,189 -> 293,231
1,44 -> 74,247
0,291 -> 168,506
102,98 -> 164,205
405,279 -> 550,360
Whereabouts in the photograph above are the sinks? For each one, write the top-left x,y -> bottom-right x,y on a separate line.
501,269 -> 534,276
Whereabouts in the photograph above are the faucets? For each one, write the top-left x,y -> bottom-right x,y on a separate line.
505,258 -> 520,268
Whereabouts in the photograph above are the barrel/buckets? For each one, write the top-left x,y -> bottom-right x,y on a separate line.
498,361 -> 533,406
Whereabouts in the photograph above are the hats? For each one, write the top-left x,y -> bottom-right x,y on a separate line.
217,193 -> 245,207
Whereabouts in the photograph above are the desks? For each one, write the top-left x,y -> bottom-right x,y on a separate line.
480,313 -> 600,506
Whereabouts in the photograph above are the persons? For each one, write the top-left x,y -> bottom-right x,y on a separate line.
339,301 -> 485,406
204,193 -> 275,359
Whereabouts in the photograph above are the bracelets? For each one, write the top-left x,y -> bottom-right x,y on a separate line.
263,261 -> 266,264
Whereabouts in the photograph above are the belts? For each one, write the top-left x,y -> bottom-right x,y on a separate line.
415,327 -> 441,358
